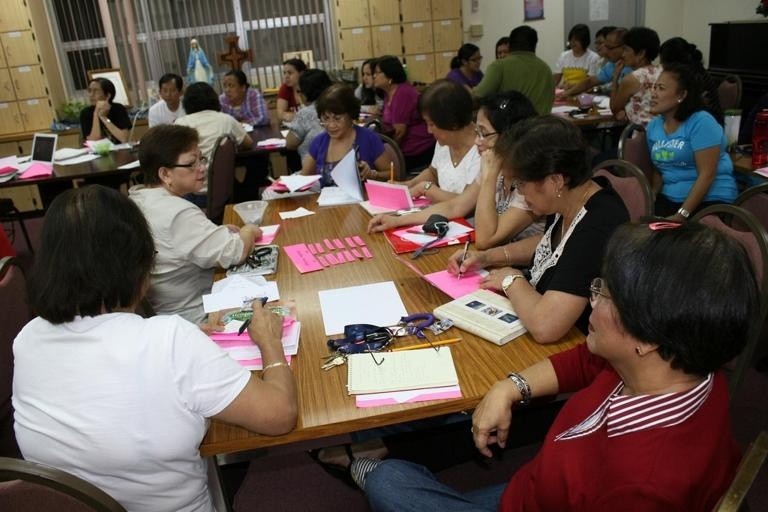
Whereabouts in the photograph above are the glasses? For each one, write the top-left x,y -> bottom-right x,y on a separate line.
472,127 -> 498,140
590,278 -> 612,300
164,156 -> 207,171
319,116 -> 349,128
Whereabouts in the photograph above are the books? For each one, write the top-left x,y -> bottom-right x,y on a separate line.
432,289 -> 527,345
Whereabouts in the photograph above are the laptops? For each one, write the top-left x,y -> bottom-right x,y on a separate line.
16,134 -> 59,174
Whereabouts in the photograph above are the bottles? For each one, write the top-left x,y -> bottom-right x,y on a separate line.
752,112 -> 768,171
723,107 -> 742,149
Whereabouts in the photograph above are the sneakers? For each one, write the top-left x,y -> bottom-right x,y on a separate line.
351,457 -> 383,493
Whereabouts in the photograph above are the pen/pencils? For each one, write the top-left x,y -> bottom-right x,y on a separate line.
387,338 -> 462,352
18,160 -> 29,164
391,161 -> 394,184
406,230 -> 438,237
458,241 -> 469,280
238,296 -> 269,336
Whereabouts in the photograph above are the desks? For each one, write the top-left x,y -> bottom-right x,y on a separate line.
0,123 -> 286,188
550,98 -> 616,126
727,141 -> 752,175
199,192 -> 586,511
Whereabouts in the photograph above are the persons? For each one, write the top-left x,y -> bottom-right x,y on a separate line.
448,25 -> 554,115
278,56 -> 436,187
9,185 -> 299,512
83,72 -> 269,205
447,113 -> 631,343
644,63 -> 737,223
316,221 -> 756,512
553,21 -> 739,168
367,79 -> 549,248
128,124 -> 263,321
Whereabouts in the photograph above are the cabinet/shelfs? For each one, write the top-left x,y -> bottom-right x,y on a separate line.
331,1 -> 464,88
0,0 -> 69,135
0,108 -> 288,216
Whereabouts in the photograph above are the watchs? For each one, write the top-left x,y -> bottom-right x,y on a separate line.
500,274 -> 524,297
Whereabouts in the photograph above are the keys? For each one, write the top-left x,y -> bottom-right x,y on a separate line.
320,350 -> 346,371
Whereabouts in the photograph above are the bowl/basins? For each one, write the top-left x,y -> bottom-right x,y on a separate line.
94,142 -> 111,156
233,201 -> 269,226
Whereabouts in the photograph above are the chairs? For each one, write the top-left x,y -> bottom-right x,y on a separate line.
724,182 -> 768,232
362,117 -> 383,132
0,458 -> 127,511
591,158 -> 655,224
0,198 -> 35,256
690,204 -> 768,408
617,121 -> 653,189
717,73 -> 743,112
205,132 -> 240,223
711,424 -> 768,512
377,132 -> 407,181
0,257 -> 33,457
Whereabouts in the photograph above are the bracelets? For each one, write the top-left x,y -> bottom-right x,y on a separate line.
677,207 -> 690,218
500,246 -> 509,268
508,371 -> 531,403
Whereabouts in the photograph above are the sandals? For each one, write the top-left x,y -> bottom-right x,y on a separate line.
307,445 -> 354,471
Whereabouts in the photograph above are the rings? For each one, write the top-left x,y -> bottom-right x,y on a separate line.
471,427 -> 474,434
